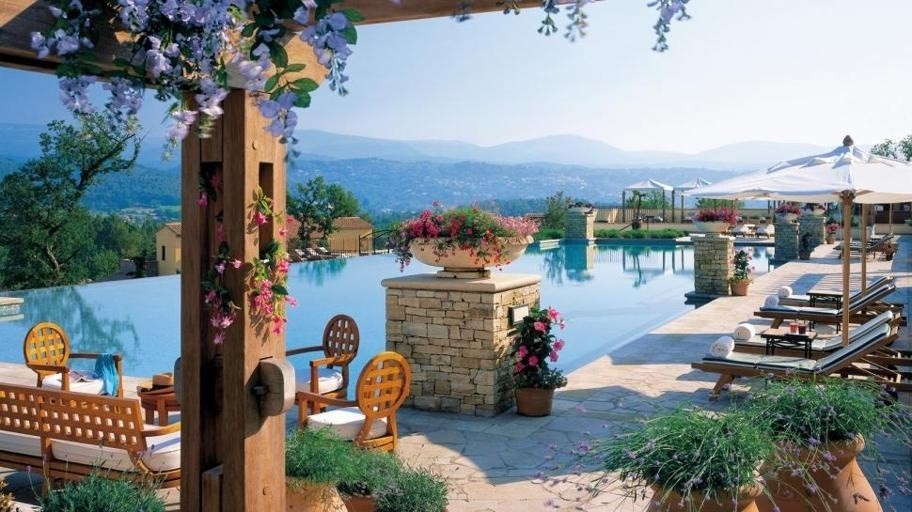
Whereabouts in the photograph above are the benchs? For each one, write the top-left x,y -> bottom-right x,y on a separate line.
1,383 -> 183,499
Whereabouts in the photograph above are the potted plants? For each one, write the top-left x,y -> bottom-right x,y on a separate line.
285,422 -> 451,512
881,243 -> 898,261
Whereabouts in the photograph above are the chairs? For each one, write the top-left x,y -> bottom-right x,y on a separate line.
23,322 -> 125,412
286,315 -> 412,457
833,233 -> 894,260
294,247 -> 340,262
691,274 -> 911,400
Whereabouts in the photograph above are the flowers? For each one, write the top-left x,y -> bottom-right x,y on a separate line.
529,363 -> 912,512
359,198 -> 540,274
693,199 -> 827,226
29,2 -> 367,168
825,223 -> 839,237
506,305 -> 569,389
191,163 -> 298,348
727,248 -> 756,288
798,232 -> 820,255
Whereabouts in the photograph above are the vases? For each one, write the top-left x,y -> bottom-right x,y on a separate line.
408,235 -> 531,279
694,221 -> 730,234
514,387 -> 554,416
799,251 -> 810,259
729,280 -> 751,297
825,236 -> 835,244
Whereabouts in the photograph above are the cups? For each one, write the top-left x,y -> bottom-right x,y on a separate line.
790,323 -> 806,334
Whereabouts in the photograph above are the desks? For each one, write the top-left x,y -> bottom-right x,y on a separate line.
141,386 -> 181,426
